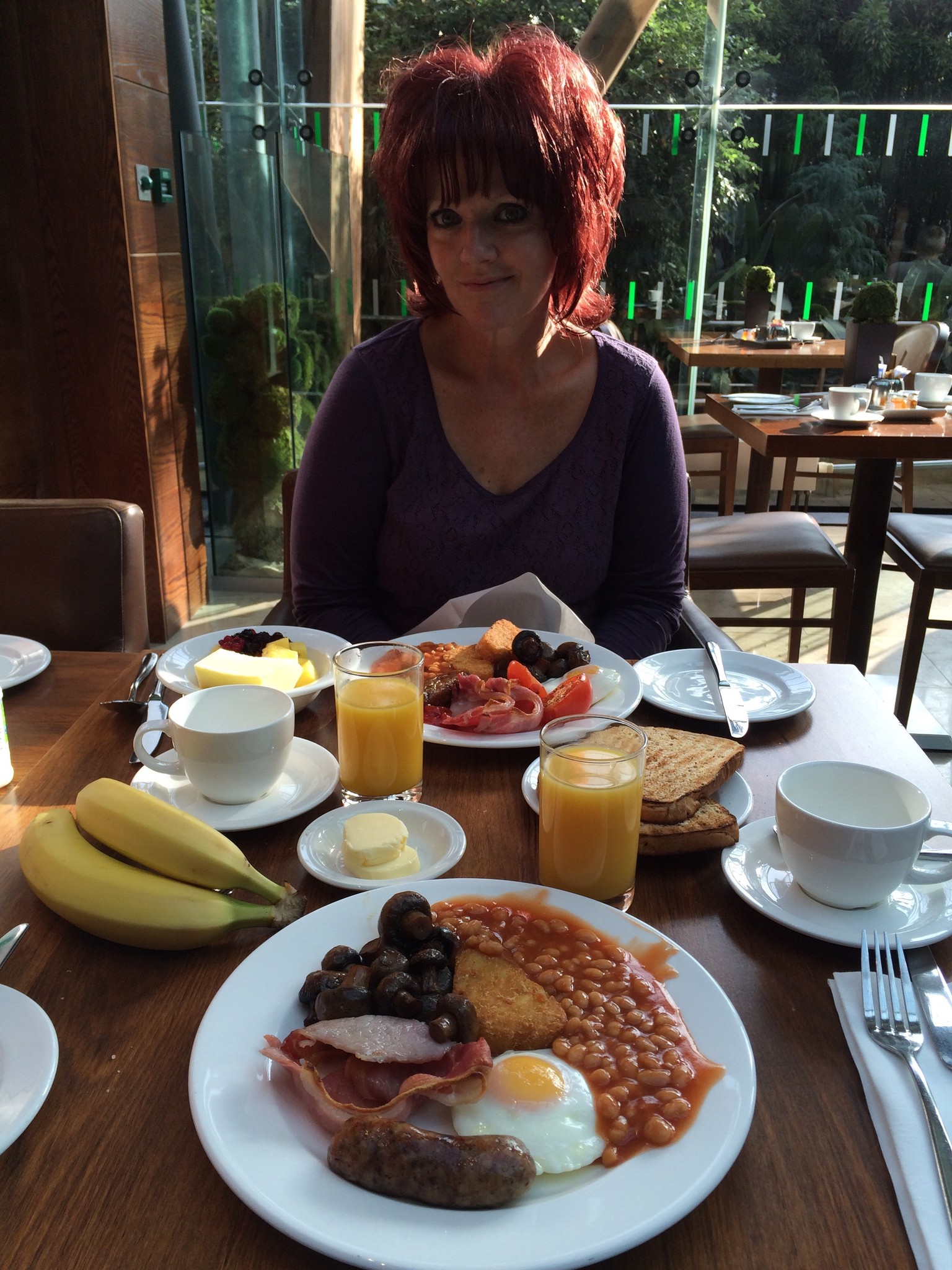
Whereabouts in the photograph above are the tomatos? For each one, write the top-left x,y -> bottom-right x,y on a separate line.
508,659 -> 548,700
540,673 -> 593,726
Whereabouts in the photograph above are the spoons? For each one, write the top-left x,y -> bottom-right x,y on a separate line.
737,399 -> 817,410
100,652 -> 158,712
129,651 -> 173,764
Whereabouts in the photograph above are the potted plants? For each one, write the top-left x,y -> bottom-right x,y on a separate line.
743,266 -> 775,332
844,279 -> 899,390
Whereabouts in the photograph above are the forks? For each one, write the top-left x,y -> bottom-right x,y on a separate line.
858,929 -> 952,1229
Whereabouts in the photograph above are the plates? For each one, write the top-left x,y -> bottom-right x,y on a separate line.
522,746 -> 755,850
789,336 -> 822,341
160,624 -> 360,714
187,879 -> 757,1267
130,734 -> 345,828
353,628 -> 642,748
870,404 -> 948,417
632,648 -> 817,722
720,816 -> 951,950
296,800 -> 466,888
1,981 -> 59,1156
728,393 -> 794,403
0,632 -> 51,690
812,409 -> 885,427
898,390 -> 952,408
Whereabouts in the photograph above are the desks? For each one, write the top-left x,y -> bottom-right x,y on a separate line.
668,336 -> 897,512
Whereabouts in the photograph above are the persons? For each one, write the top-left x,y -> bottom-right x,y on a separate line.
283,24 -> 692,664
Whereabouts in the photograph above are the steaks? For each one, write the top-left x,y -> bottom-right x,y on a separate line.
424,670 -> 469,707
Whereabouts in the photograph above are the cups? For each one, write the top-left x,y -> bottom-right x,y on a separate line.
538,712 -> 646,918
774,761 -> 951,911
330,639 -> 424,809
865,375 -> 904,407
913,373 -> 952,402
133,685 -> 296,805
828,386 -> 872,419
791,321 -> 815,338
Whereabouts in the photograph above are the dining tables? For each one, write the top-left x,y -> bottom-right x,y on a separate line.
704,387 -> 952,676
1,640 -> 952,1270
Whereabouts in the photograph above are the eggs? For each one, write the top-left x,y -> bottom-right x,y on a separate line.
451,1049 -> 606,1174
543,664 -> 621,706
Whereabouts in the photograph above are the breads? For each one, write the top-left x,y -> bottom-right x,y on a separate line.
583,723 -> 745,853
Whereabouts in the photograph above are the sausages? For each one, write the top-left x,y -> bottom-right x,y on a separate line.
328,1115 -> 538,1211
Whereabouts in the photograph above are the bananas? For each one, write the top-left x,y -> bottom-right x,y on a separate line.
21,779 -> 302,953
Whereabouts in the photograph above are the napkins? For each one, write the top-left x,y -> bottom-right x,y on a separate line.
731,403 -> 825,418
825,969 -> 952,1269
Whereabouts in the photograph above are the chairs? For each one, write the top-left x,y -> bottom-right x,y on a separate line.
2,495 -> 158,656
598,317 -> 739,515
876,510 -> 952,751
683,478 -> 854,674
780,319 -> 950,517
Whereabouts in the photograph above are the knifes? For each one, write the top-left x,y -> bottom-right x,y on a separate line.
0,922 -> 31,975
704,640 -> 750,738
905,944 -> 951,1074
